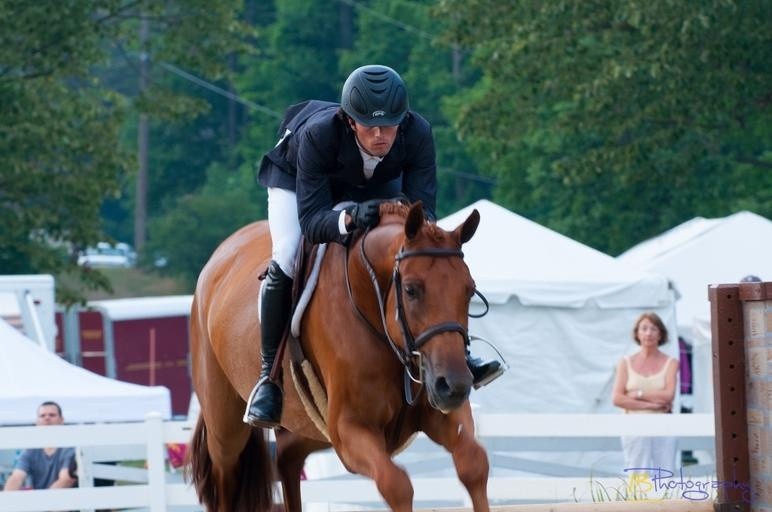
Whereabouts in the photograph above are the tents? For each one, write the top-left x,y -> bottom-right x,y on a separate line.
613,209 -> 772,500
391,199 -> 641,501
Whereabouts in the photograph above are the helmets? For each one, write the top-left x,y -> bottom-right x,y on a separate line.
342,64 -> 408,127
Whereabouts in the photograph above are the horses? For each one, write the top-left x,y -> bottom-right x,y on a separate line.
182,200 -> 490,512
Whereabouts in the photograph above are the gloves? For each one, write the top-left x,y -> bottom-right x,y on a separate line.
347,200 -> 379,228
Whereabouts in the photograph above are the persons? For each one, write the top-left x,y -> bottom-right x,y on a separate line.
612,313 -> 680,484
245,64 -> 504,428
4,402 -> 76,490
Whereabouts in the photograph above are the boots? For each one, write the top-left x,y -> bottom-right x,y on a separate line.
247,262 -> 294,423
